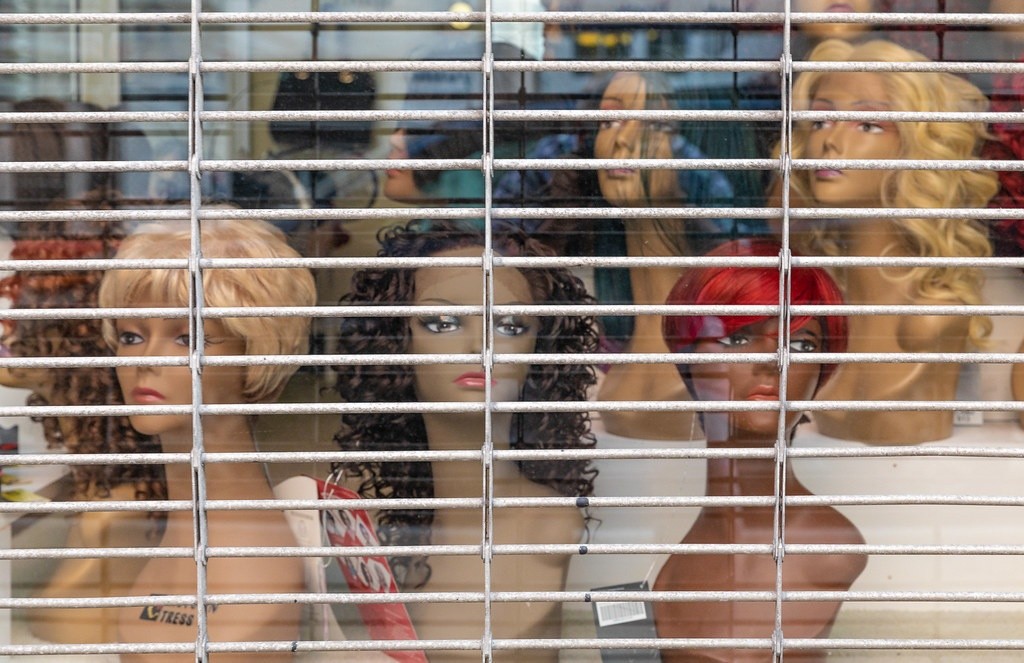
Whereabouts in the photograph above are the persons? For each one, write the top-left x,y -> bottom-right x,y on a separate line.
761,39 -> 998,447
733,0 -> 968,59
650,237 -> 868,663
332,218 -> 601,662
383,42 -> 577,204
99,202 -> 318,663
0,95 -> 153,233
0,189 -> 168,644
574,44 -> 766,442
